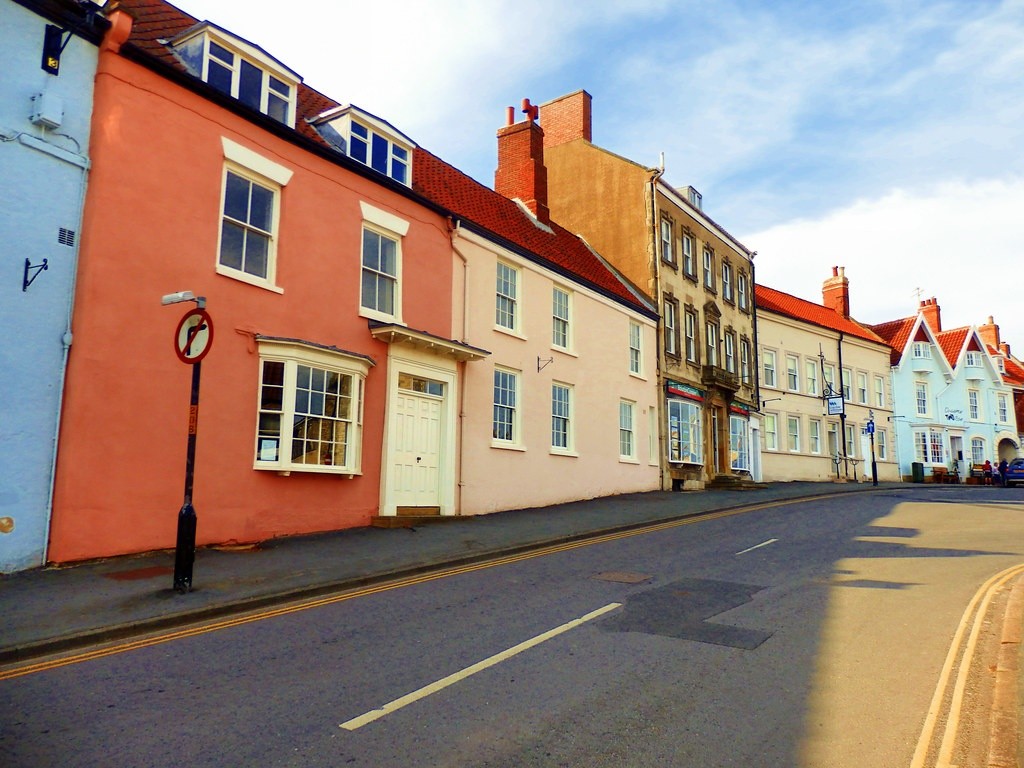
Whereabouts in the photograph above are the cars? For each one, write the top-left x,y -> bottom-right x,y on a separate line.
1003,457 -> 1024,488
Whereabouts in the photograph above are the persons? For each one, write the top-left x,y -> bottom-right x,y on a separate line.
981,458 -> 1008,487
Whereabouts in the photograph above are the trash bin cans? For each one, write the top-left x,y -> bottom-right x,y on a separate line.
911,462 -> 923,482
671,478 -> 685,491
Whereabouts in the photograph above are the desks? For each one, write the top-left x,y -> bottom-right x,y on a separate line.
931,471 -> 960,484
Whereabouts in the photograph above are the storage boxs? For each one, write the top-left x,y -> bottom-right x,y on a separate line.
967,478 -> 981,484
